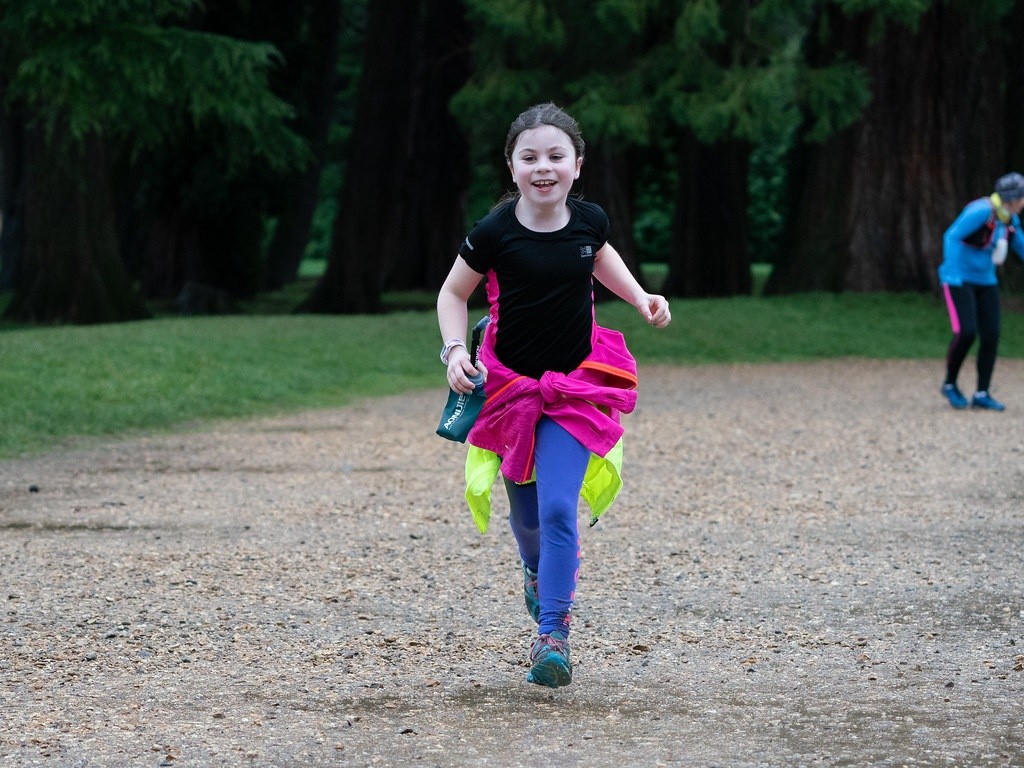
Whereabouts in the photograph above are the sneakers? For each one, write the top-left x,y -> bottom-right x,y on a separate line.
972,392 -> 1004,410
526,630 -> 572,689
940,379 -> 968,409
522,561 -> 540,626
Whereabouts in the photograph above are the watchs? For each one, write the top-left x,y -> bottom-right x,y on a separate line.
440,340 -> 468,365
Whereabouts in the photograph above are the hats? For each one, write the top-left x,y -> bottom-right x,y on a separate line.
996,171 -> 1024,201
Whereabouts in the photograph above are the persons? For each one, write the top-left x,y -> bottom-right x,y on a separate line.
938,174 -> 1024,410
437,104 -> 672,687
